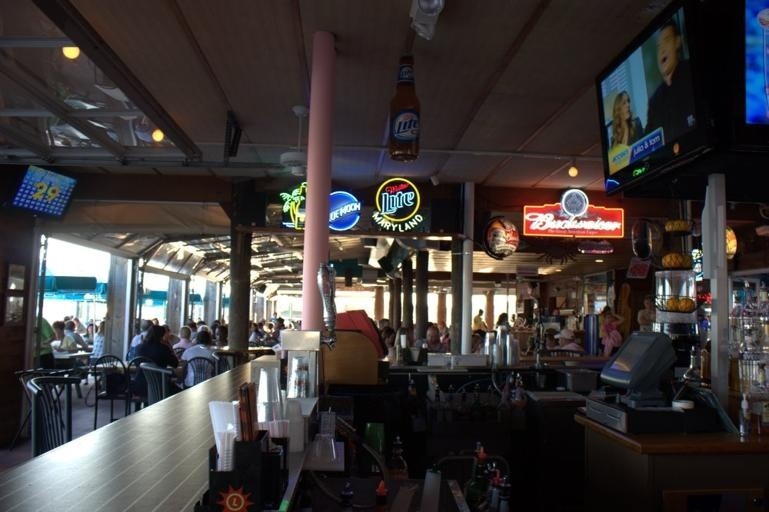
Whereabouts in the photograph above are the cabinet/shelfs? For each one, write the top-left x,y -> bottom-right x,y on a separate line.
739,392 -> 753,436
419,463 -> 443,512
389,50 -> 420,160
390,435 -> 412,479
683,348 -> 699,383
463,442 -> 511,512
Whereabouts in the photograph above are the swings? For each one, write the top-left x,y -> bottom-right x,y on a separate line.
398,328 -> 412,366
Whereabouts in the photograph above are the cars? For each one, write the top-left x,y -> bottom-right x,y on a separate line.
256,368 -> 284,431
484,325 -> 518,366
364,423 -> 385,452
286,356 -> 309,399
217,432 -> 234,473
315,434 -> 338,464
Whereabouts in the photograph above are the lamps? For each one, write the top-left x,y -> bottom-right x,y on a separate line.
93,355 -> 128,429
211,350 -> 249,377
27,376 -> 81,458
173,356 -> 215,393
139,362 -> 173,407
127,356 -> 157,415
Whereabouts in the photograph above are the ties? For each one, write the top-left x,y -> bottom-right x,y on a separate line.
600,331 -> 679,406
10,165 -> 78,220
594,0 -> 713,195
745,0 -> 769,129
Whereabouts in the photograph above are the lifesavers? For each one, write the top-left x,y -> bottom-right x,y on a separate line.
585,397 -> 693,432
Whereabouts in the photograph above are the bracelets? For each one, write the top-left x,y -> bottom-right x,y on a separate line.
83,347 -> 92,353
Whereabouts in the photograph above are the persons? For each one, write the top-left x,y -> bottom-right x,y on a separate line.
126,319 -> 230,412
599,306 -> 626,359
34,316 -> 106,404
567,311 -> 578,331
638,296 -> 656,332
379,319 -> 450,391
609,88 -> 643,148
543,327 -> 585,357
248,312 -> 286,358
471,309 -> 532,355
642,17 -> 693,156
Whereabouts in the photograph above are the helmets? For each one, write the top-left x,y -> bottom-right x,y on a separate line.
426,352 -> 487,367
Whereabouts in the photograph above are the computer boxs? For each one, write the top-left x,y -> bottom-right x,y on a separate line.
428,176 -> 440,186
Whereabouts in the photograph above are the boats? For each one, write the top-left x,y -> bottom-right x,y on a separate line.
232,178 -> 265,232
432,184 -> 463,233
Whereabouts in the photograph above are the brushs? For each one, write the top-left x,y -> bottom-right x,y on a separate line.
208,429 -> 289,512
